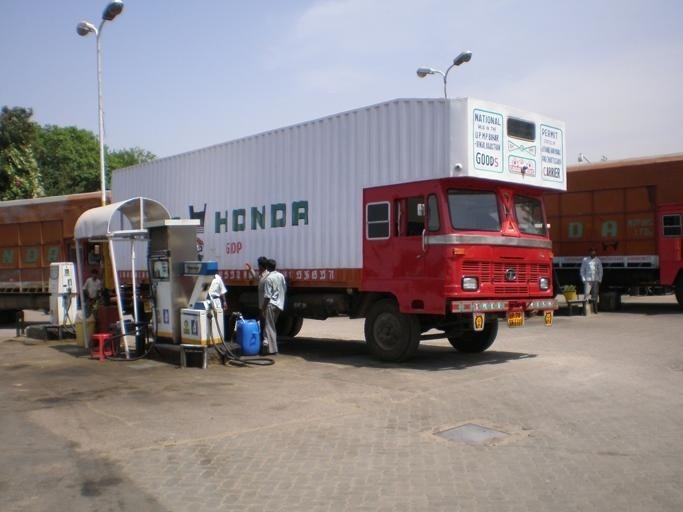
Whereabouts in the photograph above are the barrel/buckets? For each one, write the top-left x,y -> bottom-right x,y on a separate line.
74,321 -> 97,347
237,320 -> 261,354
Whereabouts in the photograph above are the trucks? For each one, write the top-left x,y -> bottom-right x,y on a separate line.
0,191 -> 110,313
538,153 -> 682,312
108,96 -> 568,360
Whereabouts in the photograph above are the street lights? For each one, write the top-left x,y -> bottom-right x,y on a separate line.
416,48 -> 473,98
75,1 -> 126,205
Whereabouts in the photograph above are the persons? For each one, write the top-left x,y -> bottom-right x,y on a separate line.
258,259 -> 288,356
206,272 -> 228,311
244,255 -> 270,338
81,268 -> 103,318
579,248 -> 603,315
